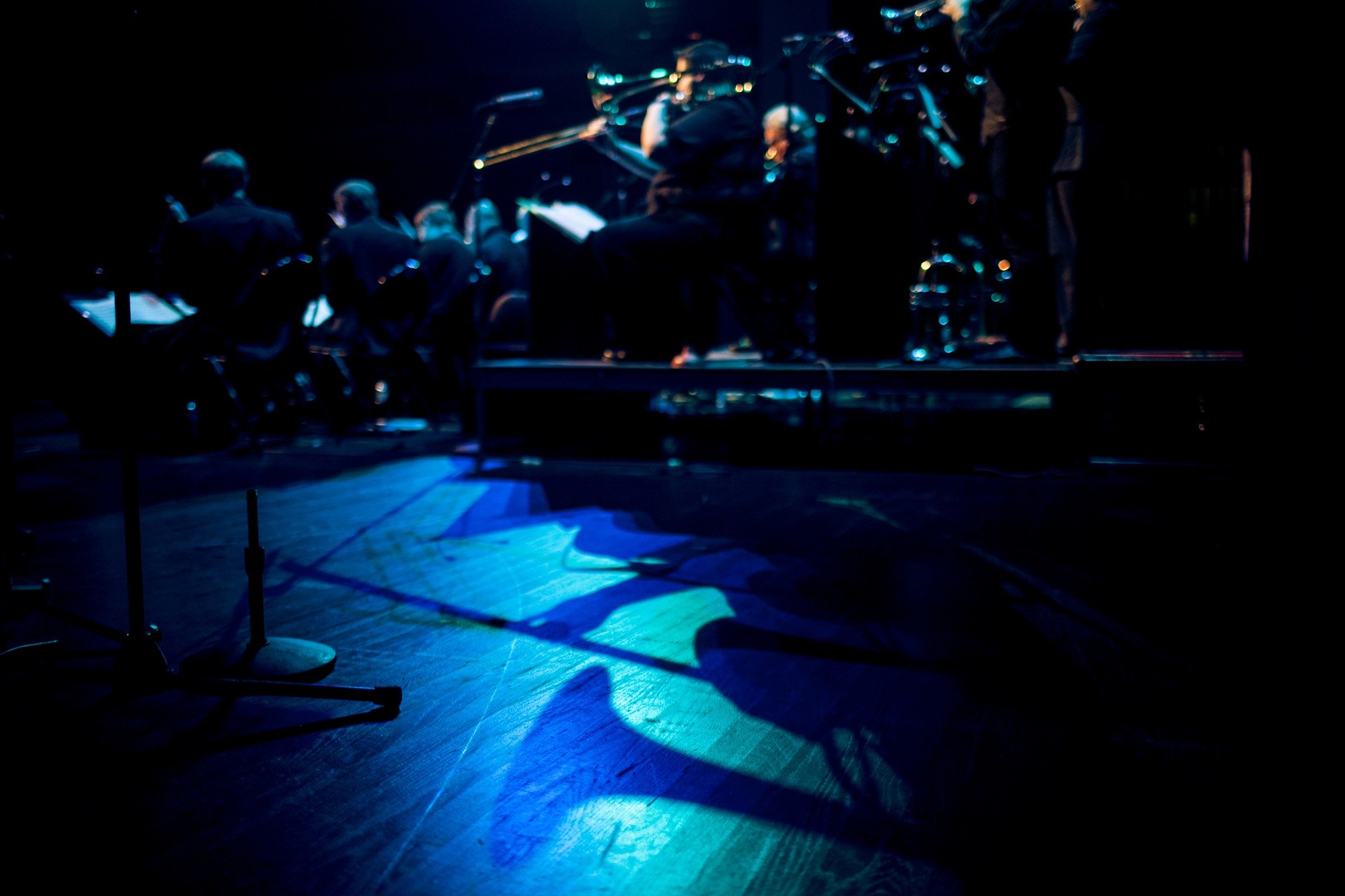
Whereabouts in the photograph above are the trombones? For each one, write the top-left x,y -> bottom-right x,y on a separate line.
469,56 -> 757,173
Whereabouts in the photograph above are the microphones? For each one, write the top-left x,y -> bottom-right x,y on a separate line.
482,87 -> 543,107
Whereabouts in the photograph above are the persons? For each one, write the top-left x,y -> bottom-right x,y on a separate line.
590,44 -> 819,366
902,0 -> 1130,365
165,151 -> 535,456
